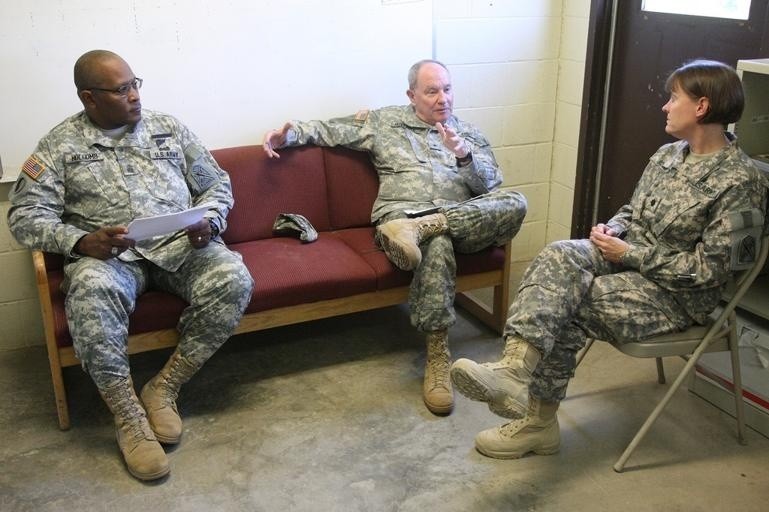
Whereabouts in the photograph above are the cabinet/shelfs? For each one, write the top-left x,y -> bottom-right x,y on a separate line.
686,58 -> 769,439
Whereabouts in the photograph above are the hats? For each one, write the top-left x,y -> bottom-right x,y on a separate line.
273,212 -> 318,243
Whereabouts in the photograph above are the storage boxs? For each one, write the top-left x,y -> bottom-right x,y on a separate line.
694,304 -> 769,403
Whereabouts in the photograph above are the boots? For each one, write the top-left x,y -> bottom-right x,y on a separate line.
96,374 -> 170,480
140,350 -> 200,444
376,213 -> 448,270
476,401 -> 561,459
423,327 -> 455,414
450,337 -> 542,419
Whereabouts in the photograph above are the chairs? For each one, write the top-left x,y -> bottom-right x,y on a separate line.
574,235 -> 768,474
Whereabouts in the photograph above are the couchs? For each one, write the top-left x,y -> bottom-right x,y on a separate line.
29,145 -> 512,430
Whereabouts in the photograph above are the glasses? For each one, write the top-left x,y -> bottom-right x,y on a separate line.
88,78 -> 145,96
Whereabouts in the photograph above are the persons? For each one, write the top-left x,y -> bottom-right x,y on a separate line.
450,58 -> 769,460
261,57 -> 530,416
6,48 -> 256,482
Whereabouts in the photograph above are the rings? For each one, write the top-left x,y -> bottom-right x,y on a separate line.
111,246 -> 117,256
197,236 -> 202,242
